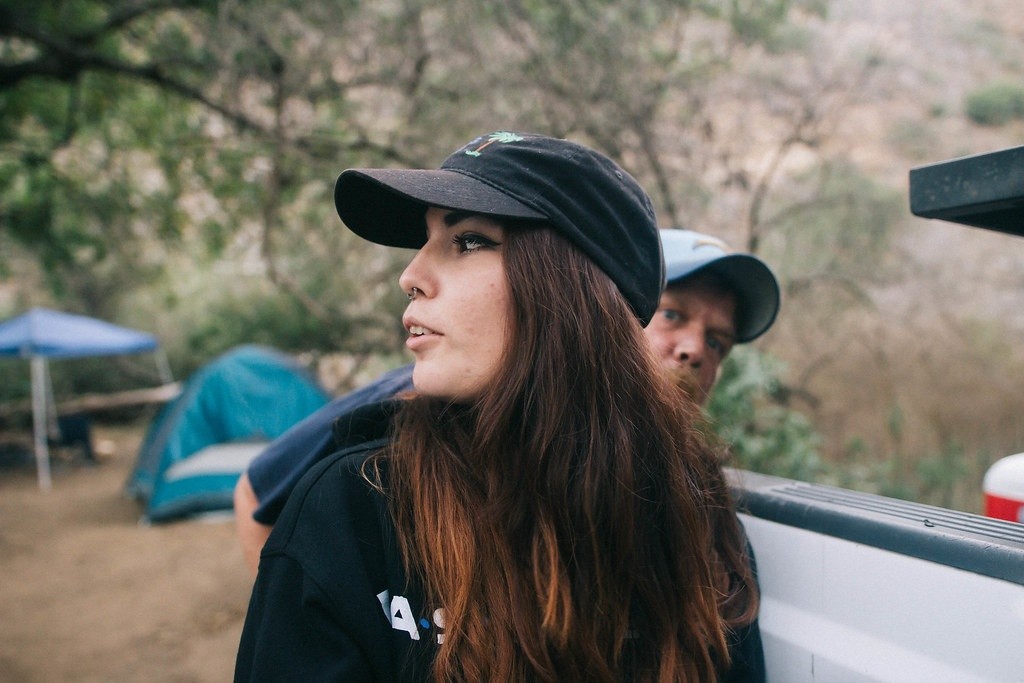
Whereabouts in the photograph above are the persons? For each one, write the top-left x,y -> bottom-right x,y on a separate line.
232,130 -> 767,682
233,227 -> 781,574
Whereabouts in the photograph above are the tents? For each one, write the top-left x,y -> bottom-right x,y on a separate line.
120,344 -> 337,527
1,300 -> 162,494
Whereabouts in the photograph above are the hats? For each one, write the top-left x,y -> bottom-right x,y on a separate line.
659,229 -> 781,343
335,131 -> 665,327
